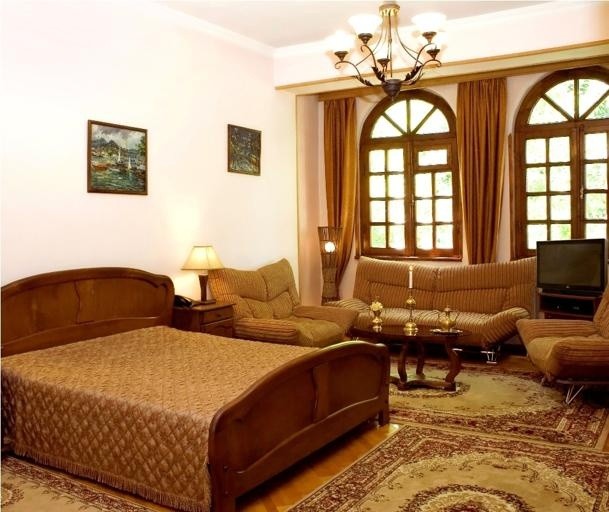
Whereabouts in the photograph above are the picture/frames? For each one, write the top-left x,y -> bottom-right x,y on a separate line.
87,118 -> 148,195
227,123 -> 264,175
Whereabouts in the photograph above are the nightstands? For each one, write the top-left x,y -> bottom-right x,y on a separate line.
174,300 -> 236,338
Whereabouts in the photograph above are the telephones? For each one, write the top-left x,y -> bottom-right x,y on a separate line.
175,294 -> 200,307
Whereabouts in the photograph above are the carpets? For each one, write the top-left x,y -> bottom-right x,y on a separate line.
287,425 -> 609,511
2,450 -> 169,512
387,358 -> 608,452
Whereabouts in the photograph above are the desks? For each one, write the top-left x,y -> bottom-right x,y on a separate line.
347,325 -> 472,391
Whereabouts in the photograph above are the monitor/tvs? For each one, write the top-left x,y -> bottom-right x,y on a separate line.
536,238 -> 608,297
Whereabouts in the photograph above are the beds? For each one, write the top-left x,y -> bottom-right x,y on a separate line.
1,266 -> 392,509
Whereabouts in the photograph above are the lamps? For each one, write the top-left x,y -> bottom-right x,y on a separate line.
179,245 -> 226,304
318,227 -> 345,307
323,0 -> 450,98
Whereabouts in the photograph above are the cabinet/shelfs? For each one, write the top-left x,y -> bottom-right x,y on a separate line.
536,290 -> 601,320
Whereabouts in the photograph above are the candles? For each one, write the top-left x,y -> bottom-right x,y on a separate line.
407,263 -> 415,289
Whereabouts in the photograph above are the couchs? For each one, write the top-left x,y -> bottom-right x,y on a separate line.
206,257 -> 360,347
516,284 -> 608,401
322,255 -> 539,365
